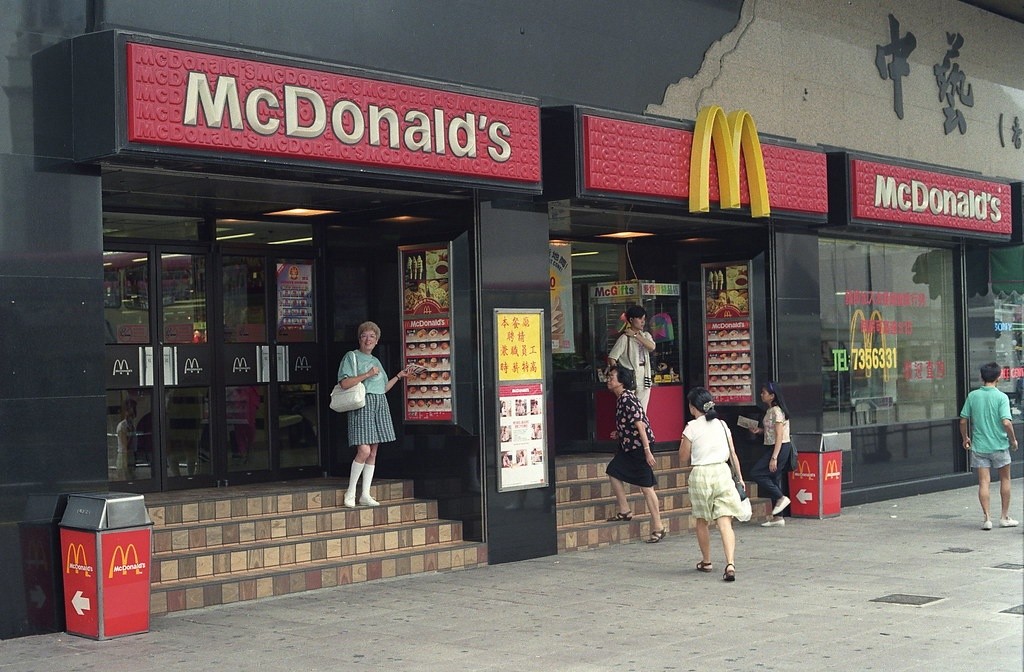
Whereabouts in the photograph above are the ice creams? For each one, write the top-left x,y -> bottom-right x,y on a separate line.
708,269 -> 723,290
407,255 -> 423,280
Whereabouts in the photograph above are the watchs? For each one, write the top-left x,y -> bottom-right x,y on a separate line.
634,332 -> 637,338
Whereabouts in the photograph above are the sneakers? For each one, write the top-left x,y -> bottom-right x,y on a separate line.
1011,407 -> 1021,414
999,515 -> 1019,526
343,490 -> 356,508
358,495 -> 380,506
980,520 -> 992,529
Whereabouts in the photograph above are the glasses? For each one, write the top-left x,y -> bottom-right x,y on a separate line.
359,333 -> 377,341
607,375 -> 614,379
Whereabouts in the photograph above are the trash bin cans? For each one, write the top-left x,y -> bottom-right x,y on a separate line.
790,431 -> 852,518
58,492 -> 154,641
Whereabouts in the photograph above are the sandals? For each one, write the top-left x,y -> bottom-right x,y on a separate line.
646,527 -> 666,543
697,561 -> 712,572
722,564 -> 735,581
608,510 -> 632,521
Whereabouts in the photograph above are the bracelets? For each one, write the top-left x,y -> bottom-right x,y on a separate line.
396,375 -> 400,380
644,447 -> 650,450
771,457 -> 777,460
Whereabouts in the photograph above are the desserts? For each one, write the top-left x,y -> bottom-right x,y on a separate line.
709,329 -> 750,393
408,329 -> 449,407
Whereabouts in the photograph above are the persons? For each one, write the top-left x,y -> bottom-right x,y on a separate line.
338,321 -> 414,508
678,387 -> 746,581
605,365 -> 666,543
749,381 -> 792,527
115,399 -> 139,481
959,362 -> 1019,529
607,305 -> 656,416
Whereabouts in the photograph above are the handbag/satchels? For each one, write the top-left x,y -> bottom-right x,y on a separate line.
328,350 -> 365,412
781,435 -> 798,471
629,370 -> 636,390
732,476 -> 748,501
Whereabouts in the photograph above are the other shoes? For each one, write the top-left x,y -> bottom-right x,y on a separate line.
199,452 -> 211,462
771,496 -> 791,516
761,519 -> 785,527
